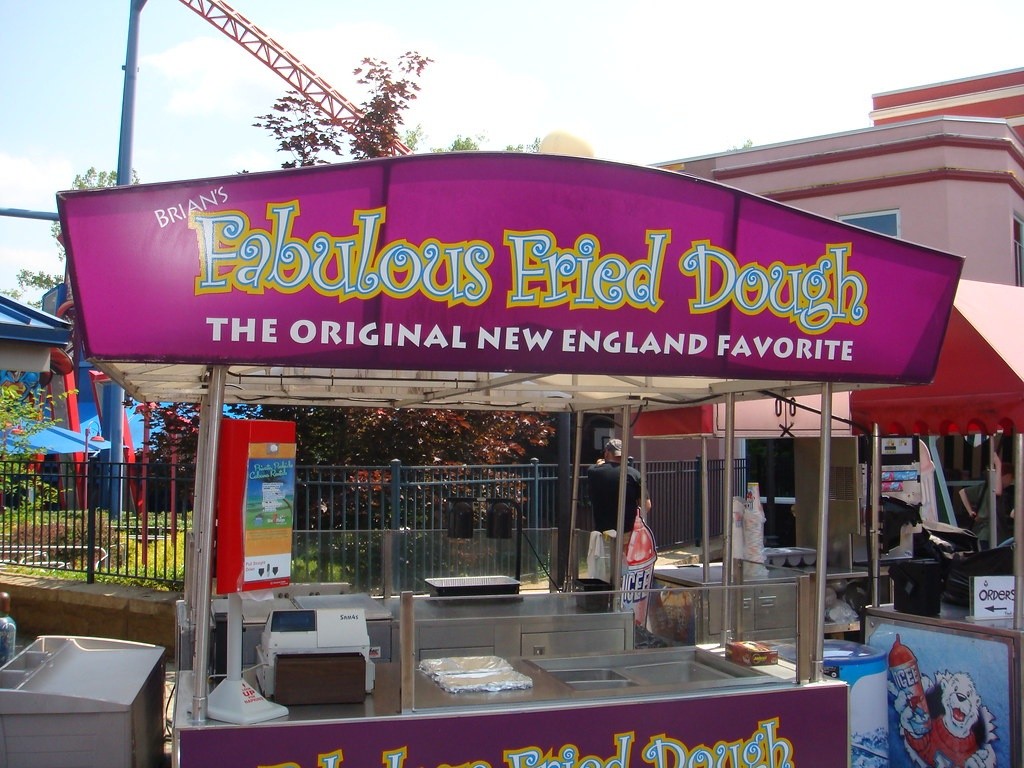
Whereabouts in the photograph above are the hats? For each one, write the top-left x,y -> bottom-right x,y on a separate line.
605,438 -> 622,457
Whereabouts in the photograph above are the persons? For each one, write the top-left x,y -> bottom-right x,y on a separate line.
588,439 -> 651,586
959,465 -> 1015,551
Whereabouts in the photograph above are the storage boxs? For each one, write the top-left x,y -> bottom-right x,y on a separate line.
724,641 -> 778,667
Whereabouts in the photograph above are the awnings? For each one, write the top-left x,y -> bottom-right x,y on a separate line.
851,277 -> 1024,437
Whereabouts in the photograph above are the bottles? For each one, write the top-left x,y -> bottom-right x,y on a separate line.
741,483 -> 765,564
